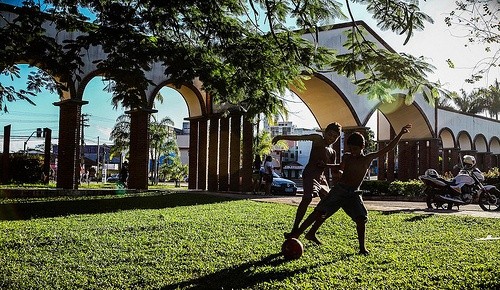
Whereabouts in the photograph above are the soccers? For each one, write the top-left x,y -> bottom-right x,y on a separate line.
282,238 -> 303,259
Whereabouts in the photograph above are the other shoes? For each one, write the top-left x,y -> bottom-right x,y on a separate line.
253,189 -> 257,195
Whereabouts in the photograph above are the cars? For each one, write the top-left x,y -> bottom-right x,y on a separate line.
252,166 -> 298,196
107,174 -> 120,183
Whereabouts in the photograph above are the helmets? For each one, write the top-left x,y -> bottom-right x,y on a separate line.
463,154 -> 477,169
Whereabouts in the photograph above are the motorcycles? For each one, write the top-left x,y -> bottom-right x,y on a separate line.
417,166 -> 500,212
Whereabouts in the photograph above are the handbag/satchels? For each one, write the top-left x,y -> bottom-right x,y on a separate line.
262,173 -> 272,182
252,173 -> 262,180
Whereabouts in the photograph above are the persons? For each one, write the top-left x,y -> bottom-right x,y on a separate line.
272,121 -> 410,256
253,154 -> 274,196
120,160 -> 129,186
